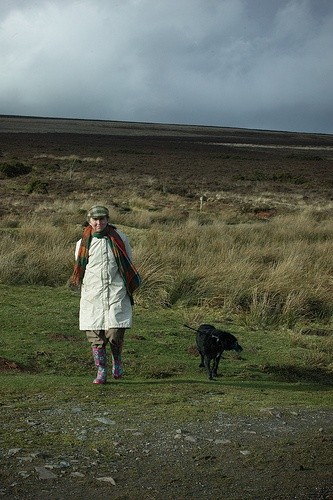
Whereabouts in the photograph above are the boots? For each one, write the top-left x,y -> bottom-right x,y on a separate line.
109,340 -> 124,379
92,347 -> 107,384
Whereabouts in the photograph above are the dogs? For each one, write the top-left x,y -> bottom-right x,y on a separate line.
196,323 -> 244,381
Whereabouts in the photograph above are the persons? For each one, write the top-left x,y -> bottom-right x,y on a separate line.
73,205 -> 142,385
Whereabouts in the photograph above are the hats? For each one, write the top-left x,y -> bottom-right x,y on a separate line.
87,206 -> 109,218
